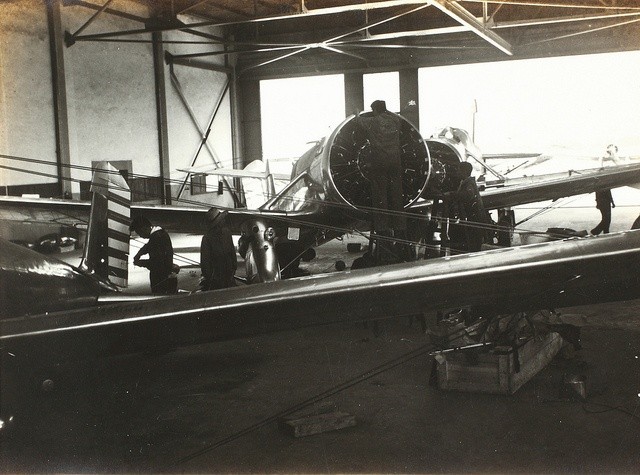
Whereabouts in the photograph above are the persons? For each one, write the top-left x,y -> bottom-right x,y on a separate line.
498,211 -> 504,222
443,161 -> 489,251
506,210 -> 514,240
129,217 -> 180,295
351,253 -> 373,270
590,186 -> 615,235
200,207 -> 238,292
498,217 -> 510,247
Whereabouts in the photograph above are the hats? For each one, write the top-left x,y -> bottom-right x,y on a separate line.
201,208 -> 228,229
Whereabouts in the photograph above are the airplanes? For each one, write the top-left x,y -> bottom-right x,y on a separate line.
420,100 -> 549,200
0,161 -> 169,318
0,100 -> 640,282
